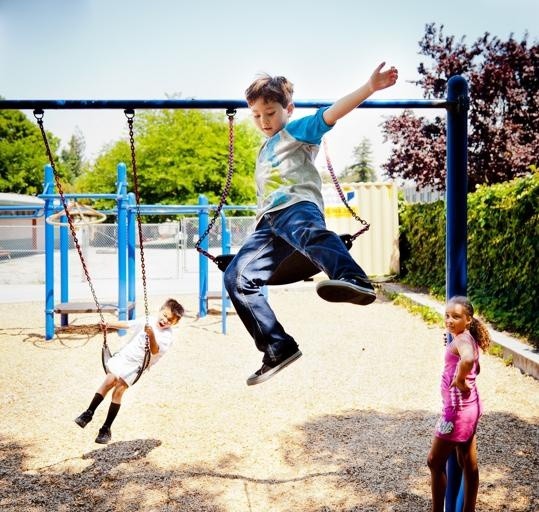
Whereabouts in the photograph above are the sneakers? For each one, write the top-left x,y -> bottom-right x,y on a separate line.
247,348 -> 302,385
75,411 -> 92,427
315,276 -> 376,305
95,428 -> 111,444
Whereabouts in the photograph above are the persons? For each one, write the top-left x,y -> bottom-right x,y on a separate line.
425,294 -> 491,510
222,59 -> 399,386
73,297 -> 185,445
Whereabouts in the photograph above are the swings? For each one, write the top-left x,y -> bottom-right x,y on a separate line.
34,109 -> 150,384
195,109 -> 370,284
